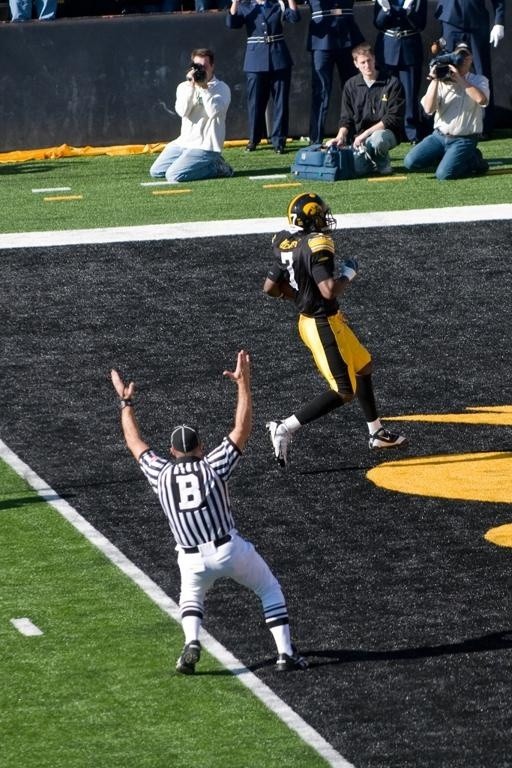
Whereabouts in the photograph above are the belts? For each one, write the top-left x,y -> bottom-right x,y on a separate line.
178,534 -> 233,554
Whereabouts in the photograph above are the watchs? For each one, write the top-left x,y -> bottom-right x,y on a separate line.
120,398 -> 133,409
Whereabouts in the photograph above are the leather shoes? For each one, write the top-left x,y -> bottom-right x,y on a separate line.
244,144 -> 258,152
274,145 -> 285,155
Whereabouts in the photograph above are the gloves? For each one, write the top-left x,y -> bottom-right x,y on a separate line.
488,23 -> 506,49
377,0 -> 392,12
402,0 -> 416,11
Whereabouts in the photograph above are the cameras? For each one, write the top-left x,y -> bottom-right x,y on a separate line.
190,64 -> 207,82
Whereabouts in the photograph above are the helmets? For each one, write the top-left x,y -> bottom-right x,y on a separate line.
286,191 -> 333,233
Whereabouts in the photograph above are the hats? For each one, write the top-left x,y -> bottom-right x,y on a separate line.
169,422 -> 201,453
454,41 -> 472,56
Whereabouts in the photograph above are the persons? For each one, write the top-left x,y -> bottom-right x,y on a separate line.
8,0 -> 62,25
262,191 -> 407,465
112,349 -> 311,673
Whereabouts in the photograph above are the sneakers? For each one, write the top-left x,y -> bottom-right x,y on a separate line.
174,639 -> 201,675
377,162 -> 394,175
263,418 -> 293,468
368,428 -> 411,451
217,156 -> 236,179
411,138 -> 421,146
474,147 -> 491,172
274,644 -> 311,672
